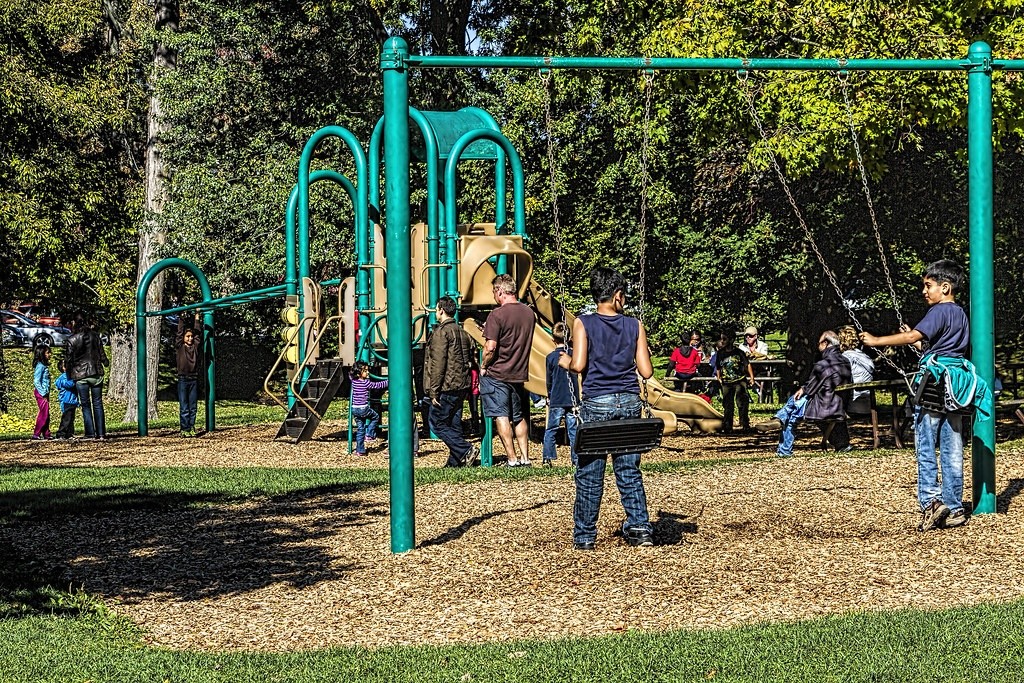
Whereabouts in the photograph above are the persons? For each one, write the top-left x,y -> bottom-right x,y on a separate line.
348,361 -> 388,456
65,311 -> 109,440
467,361 -> 481,436
543,322 -> 580,468
558,267 -> 654,550
666,331 -> 722,401
480,274 -> 536,467
423,297 -> 480,468
177,308 -> 202,436
532,394 -> 549,409
859,260 -> 970,532
32,345 -> 54,439
383,406 -> 419,459
739,327 -> 768,404
755,330 -> 853,457
55,359 -> 80,440
815,326 -> 874,453
715,329 -> 756,432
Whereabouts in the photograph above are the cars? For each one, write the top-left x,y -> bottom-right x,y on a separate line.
0,305 -> 111,347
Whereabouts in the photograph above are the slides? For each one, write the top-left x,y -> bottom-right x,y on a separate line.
519,273 -> 726,434
447,260 -> 677,435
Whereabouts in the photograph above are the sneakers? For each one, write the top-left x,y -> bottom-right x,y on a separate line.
574,541 -> 594,551
613,525 -> 653,546
917,501 -> 950,533
180,428 -> 196,437
944,509 -> 967,529
542,458 -> 552,468
464,443 -> 480,468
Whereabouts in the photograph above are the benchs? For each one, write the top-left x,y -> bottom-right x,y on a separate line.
664,374 -> 785,403
795,405 -> 893,449
955,397 -> 1024,428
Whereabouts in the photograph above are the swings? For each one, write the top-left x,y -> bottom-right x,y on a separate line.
741,70 -> 977,417
539,68 -> 665,457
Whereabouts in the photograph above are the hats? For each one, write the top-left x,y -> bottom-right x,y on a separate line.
743,327 -> 757,335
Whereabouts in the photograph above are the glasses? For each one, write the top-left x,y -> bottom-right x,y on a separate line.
817,339 -> 828,347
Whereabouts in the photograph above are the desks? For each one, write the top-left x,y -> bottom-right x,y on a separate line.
689,358 -> 790,403
835,379 -> 912,449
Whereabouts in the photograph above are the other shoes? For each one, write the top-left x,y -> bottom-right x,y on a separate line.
755,417 -> 781,431
78,434 -> 95,441
44,435 -> 53,439
32,434 -> 43,440
833,444 -> 853,454
356,451 -> 365,456
725,429 -> 738,436
495,460 -> 531,469
364,435 -> 377,442
97,435 -> 105,441
743,425 -> 752,431
534,398 -> 551,411
414,453 -> 418,459
